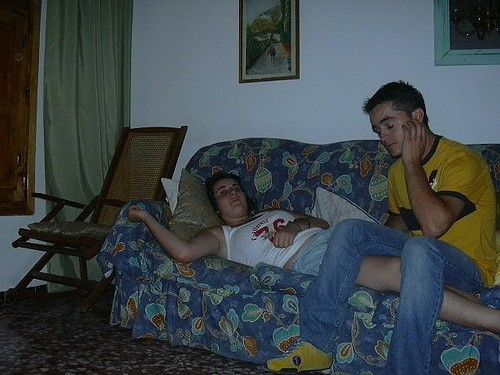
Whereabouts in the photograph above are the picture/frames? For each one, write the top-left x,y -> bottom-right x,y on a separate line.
433,0 -> 500,66
238,0 -> 301,84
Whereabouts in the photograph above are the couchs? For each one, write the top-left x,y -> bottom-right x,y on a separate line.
97,137 -> 500,375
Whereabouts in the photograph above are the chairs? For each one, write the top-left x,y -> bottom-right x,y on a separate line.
11,124 -> 189,314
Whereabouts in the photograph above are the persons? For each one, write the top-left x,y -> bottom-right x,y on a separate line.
265,81 -> 497,375
127,172 -> 500,334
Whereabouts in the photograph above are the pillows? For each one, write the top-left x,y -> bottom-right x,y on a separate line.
167,167 -> 225,242
161,177 -> 180,213
309,186 -> 383,225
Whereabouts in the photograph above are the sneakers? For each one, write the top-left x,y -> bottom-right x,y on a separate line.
264,342 -> 332,371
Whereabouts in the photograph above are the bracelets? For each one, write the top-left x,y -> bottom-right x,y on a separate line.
299,217 -> 310,229
